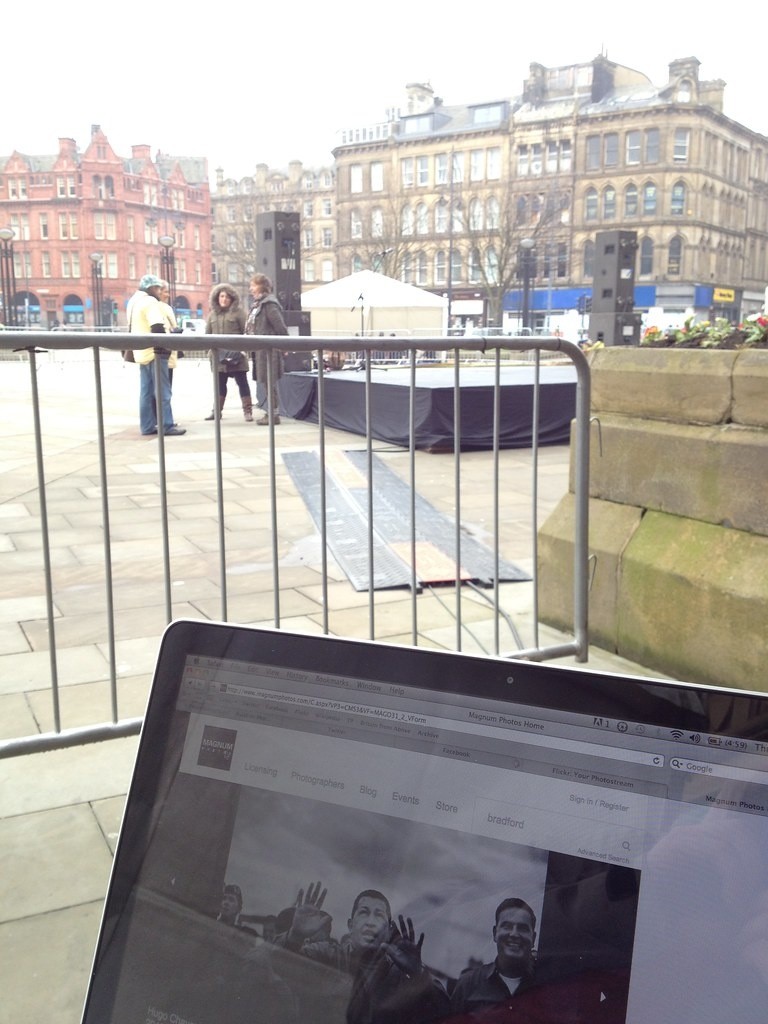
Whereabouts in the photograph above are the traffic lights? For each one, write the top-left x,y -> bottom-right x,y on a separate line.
576,295 -> 592,313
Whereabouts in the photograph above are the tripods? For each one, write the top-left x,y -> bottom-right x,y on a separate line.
342,254 -> 388,372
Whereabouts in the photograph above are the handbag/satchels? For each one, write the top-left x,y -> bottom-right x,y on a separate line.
219,350 -> 241,366
121,347 -> 135,363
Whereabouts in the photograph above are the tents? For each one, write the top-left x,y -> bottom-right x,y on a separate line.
301,269 -> 448,364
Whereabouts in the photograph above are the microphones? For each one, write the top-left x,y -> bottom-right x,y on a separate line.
378,247 -> 394,255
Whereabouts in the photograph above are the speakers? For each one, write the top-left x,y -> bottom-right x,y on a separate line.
591,231 -> 637,312
588,313 -> 641,346
252,311 -> 311,380
254,212 -> 301,310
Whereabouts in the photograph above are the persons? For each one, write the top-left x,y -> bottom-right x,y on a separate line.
245,274 -> 289,425
203,283 -> 255,422
218,884 -> 538,1023
126,274 -> 187,436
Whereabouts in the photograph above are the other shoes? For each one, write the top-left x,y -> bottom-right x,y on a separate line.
163,425 -> 186,435
257,411 -> 281,425
143,427 -> 159,435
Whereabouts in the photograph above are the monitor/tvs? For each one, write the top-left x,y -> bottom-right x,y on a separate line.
79,619 -> 768,1024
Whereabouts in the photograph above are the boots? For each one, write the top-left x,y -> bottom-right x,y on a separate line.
205,395 -> 226,419
240,396 -> 254,422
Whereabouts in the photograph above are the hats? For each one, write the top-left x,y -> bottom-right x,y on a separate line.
224,884 -> 242,907
139,275 -> 162,290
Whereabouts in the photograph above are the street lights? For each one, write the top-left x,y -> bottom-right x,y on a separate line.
89,253 -> 104,332
158,233 -> 177,326
0,228 -> 18,328
518,238 -> 536,327
